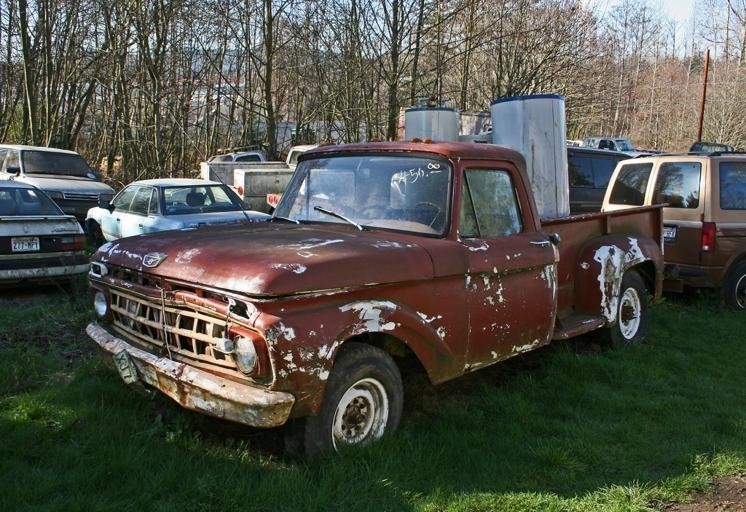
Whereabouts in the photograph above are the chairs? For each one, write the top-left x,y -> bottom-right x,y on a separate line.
187,193 -> 209,206
17,202 -> 41,214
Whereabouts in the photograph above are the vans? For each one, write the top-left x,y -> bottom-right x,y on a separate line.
601,150 -> 746,312
568,146 -> 633,217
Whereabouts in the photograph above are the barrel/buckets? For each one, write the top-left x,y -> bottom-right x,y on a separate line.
489,94 -> 570,221
404,105 -> 458,142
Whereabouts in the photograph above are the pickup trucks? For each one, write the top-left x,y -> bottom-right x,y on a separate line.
84,138 -> 670,466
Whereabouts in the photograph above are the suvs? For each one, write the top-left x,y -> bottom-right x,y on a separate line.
1,143 -> 117,229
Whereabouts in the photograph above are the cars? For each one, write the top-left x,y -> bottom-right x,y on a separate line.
84,177 -> 274,252
0,180 -> 91,279
